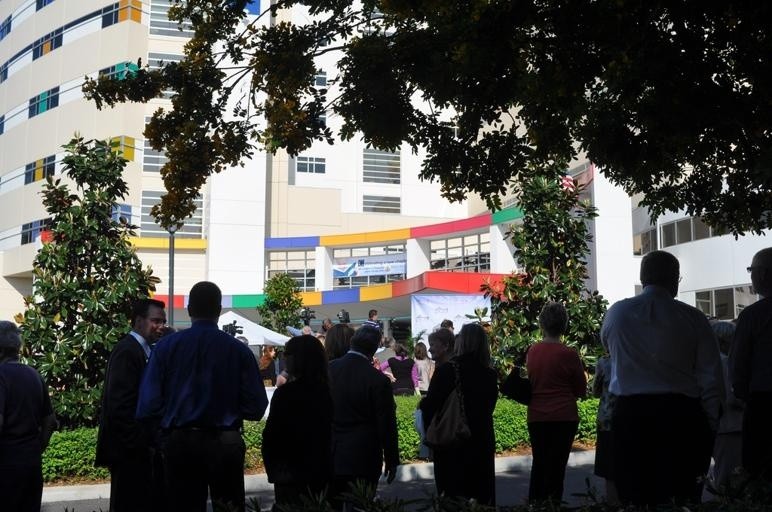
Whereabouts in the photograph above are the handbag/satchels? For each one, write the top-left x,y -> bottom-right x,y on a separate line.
423,359 -> 471,450
500,343 -> 531,405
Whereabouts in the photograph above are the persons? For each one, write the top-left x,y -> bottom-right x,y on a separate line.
521,247 -> 771,512
2,244 -> 508,511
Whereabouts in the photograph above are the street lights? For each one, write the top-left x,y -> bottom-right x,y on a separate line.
162,213 -> 186,328
